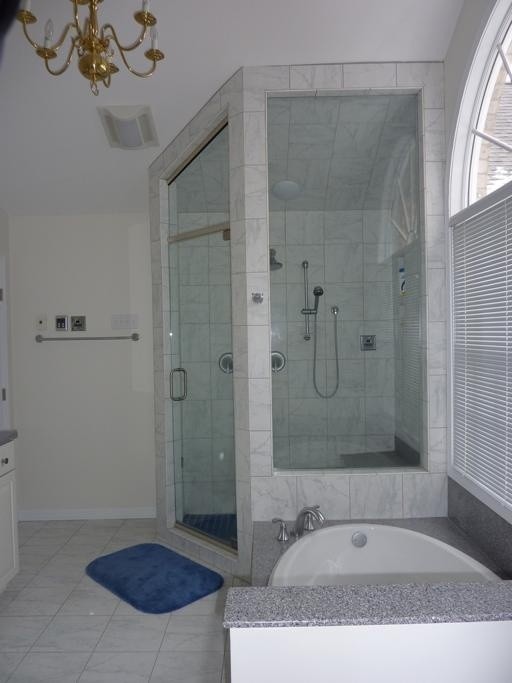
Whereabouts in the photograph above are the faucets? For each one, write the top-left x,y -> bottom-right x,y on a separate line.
293,506 -> 329,539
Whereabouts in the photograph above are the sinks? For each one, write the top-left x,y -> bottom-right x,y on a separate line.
268,521 -> 507,585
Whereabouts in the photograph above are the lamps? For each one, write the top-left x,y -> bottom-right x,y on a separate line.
95,101 -> 160,150
271,176 -> 302,201
13,0 -> 165,94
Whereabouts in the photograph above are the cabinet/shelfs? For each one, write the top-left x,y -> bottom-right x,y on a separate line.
0,424 -> 19,598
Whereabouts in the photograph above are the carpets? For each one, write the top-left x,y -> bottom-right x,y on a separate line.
84,541 -> 225,613
